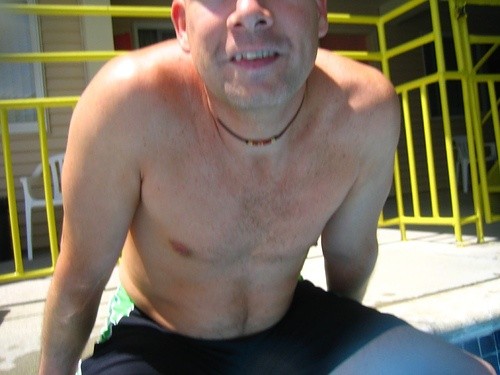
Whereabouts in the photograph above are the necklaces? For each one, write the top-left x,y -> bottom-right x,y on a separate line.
216,92 -> 306,146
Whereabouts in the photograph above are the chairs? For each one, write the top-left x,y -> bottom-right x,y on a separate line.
452,135 -> 496,193
19,153 -> 64,261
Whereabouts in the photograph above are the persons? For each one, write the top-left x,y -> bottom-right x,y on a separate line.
38,0 -> 497,375
